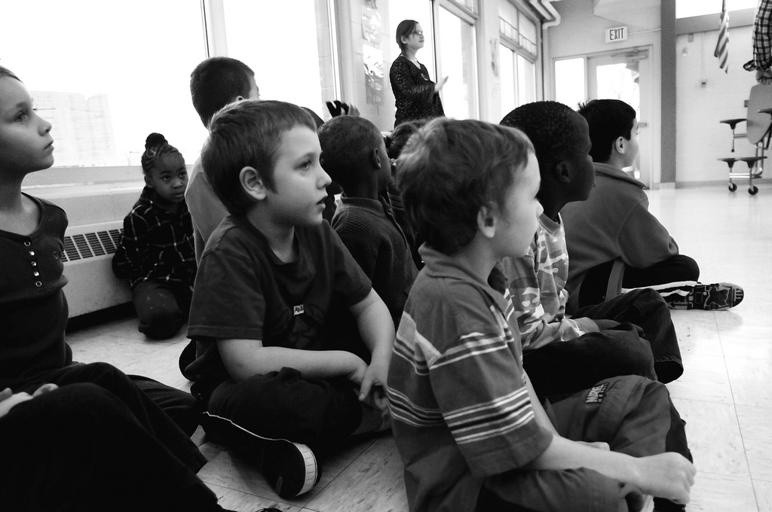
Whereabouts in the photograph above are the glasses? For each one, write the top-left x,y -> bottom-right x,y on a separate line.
410,31 -> 423,35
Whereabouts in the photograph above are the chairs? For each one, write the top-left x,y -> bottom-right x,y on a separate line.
717,84 -> 772,195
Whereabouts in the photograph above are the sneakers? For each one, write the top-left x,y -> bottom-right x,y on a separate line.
260,442 -> 323,501
665,282 -> 744,310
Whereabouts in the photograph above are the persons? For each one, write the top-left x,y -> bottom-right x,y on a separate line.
389,20 -> 449,128
557,102 -> 748,314
389,118 -> 698,511
498,102 -> 685,399
111,132 -> 196,341
1,67 -> 230,512
184,57 -> 260,266
177,96 -> 397,501
317,116 -> 422,324
299,100 -> 361,224
383,120 -> 419,158
